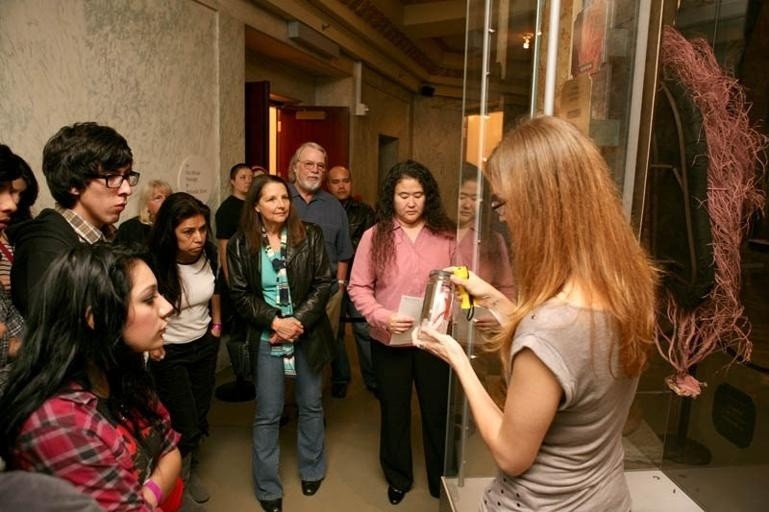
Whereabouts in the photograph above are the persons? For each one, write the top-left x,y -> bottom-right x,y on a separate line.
412,114 -> 666,512
444,162 -> 515,487
1,121 -> 458,512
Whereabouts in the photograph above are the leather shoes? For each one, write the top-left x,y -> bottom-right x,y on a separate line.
388,484 -> 406,504
333,381 -> 349,396
259,498 -> 282,512
367,378 -> 378,398
301,479 -> 323,495
428,481 -> 441,498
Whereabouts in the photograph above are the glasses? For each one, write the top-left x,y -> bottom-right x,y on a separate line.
93,171 -> 140,188
491,200 -> 506,213
298,159 -> 326,172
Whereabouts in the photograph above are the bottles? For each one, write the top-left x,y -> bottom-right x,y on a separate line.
417,268 -> 457,343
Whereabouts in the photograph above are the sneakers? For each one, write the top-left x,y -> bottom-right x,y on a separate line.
189,483 -> 210,503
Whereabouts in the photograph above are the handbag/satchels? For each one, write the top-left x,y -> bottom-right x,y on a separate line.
225,337 -> 250,380
